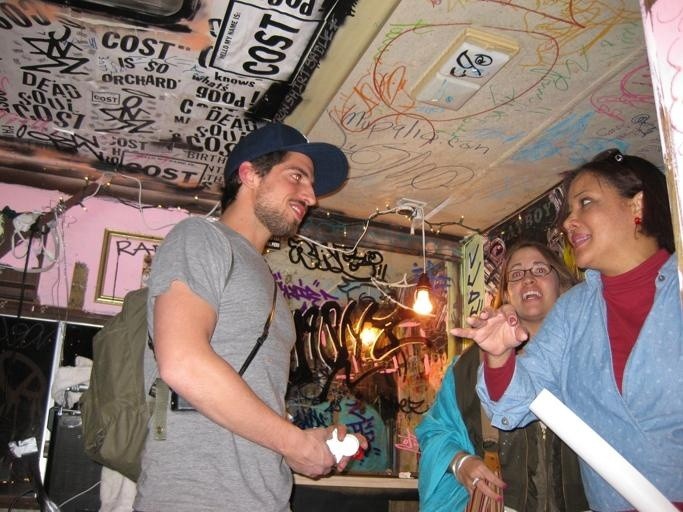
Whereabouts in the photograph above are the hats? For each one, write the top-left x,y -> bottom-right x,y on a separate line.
224,124 -> 348,197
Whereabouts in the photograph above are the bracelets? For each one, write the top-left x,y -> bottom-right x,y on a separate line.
451,455 -> 472,479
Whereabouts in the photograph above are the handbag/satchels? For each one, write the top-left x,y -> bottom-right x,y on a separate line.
78,288 -> 150,482
467,438 -> 505,512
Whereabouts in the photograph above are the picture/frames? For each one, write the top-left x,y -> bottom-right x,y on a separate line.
93,228 -> 165,306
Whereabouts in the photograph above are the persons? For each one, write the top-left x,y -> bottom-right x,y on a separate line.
415,241 -> 588,512
130,125 -> 367,512
447,146 -> 682,512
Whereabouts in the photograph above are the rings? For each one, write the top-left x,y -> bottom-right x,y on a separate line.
471,477 -> 479,486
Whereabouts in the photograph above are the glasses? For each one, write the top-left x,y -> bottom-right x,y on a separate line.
594,147 -> 626,167
505,264 -> 552,282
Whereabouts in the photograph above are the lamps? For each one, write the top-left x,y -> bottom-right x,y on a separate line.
358,296 -> 377,350
413,211 -> 434,315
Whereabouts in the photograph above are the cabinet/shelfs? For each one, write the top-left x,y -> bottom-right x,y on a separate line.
0,295 -> 118,510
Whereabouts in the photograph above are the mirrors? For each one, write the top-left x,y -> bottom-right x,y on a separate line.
263,211 -> 463,479
